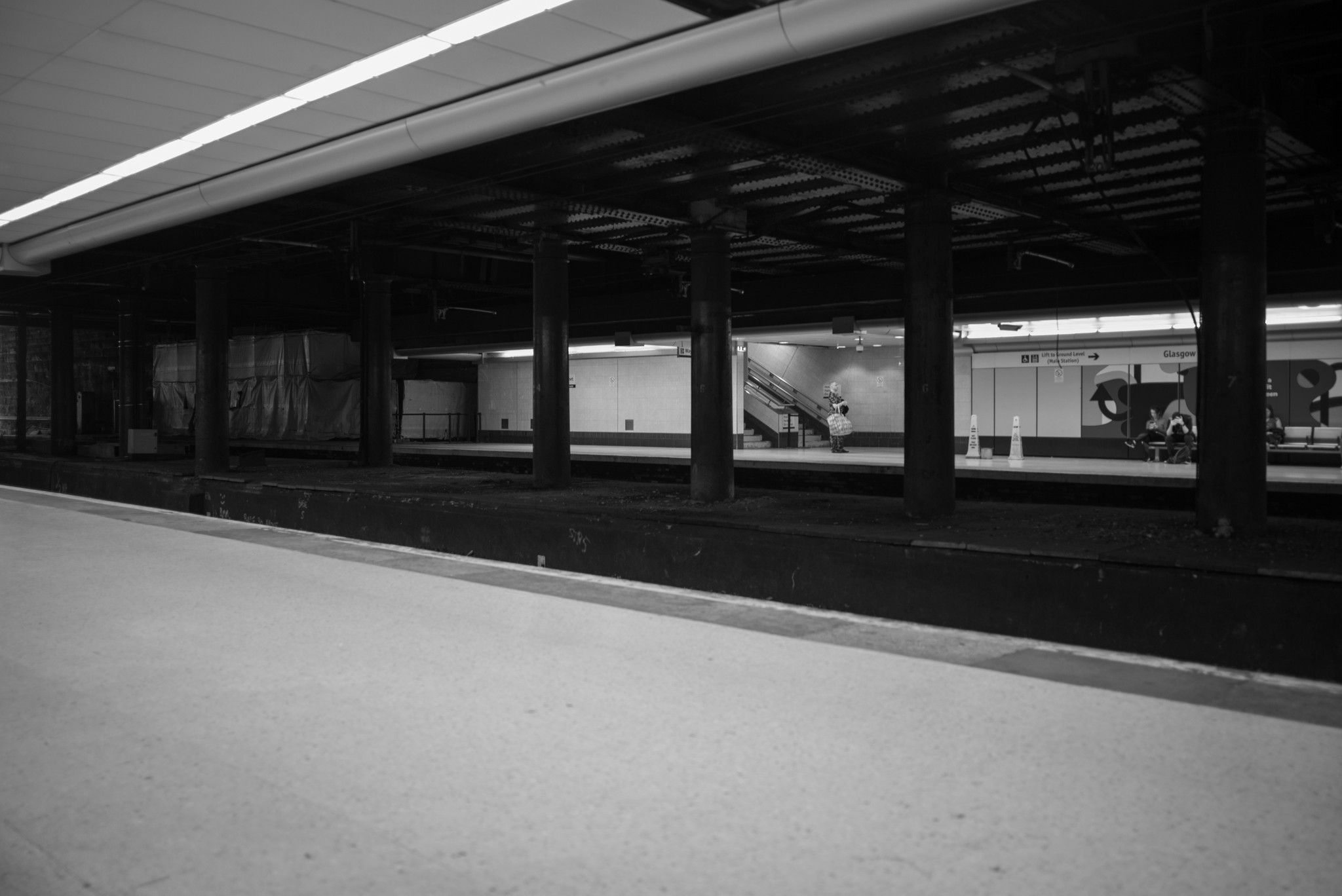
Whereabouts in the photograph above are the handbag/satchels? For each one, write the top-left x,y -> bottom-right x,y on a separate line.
826,404 -> 853,436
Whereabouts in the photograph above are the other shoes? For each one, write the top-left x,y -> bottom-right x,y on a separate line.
1143,457 -> 1150,463
1164,459 -> 1173,464
1183,458 -> 1192,464
832,450 -> 842,453
840,449 -> 849,453
1125,441 -> 1135,448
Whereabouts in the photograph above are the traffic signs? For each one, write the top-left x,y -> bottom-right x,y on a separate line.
1041,349 -> 1100,366
678,346 -> 692,356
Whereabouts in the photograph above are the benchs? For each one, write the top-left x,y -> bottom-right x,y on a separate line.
1148,425 -> 1342,462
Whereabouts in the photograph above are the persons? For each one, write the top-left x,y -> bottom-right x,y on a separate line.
1266,404 -> 1283,448
1163,412 -> 1192,464
1124,407 -> 1167,462
828,382 -> 849,453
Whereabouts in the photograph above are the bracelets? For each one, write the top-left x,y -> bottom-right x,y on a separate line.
1182,424 -> 1185,426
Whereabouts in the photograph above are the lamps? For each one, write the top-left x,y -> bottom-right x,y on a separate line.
855,336 -> 863,353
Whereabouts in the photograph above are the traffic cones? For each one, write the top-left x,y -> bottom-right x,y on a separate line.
1007,415 -> 1025,461
965,414 -> 981,458
